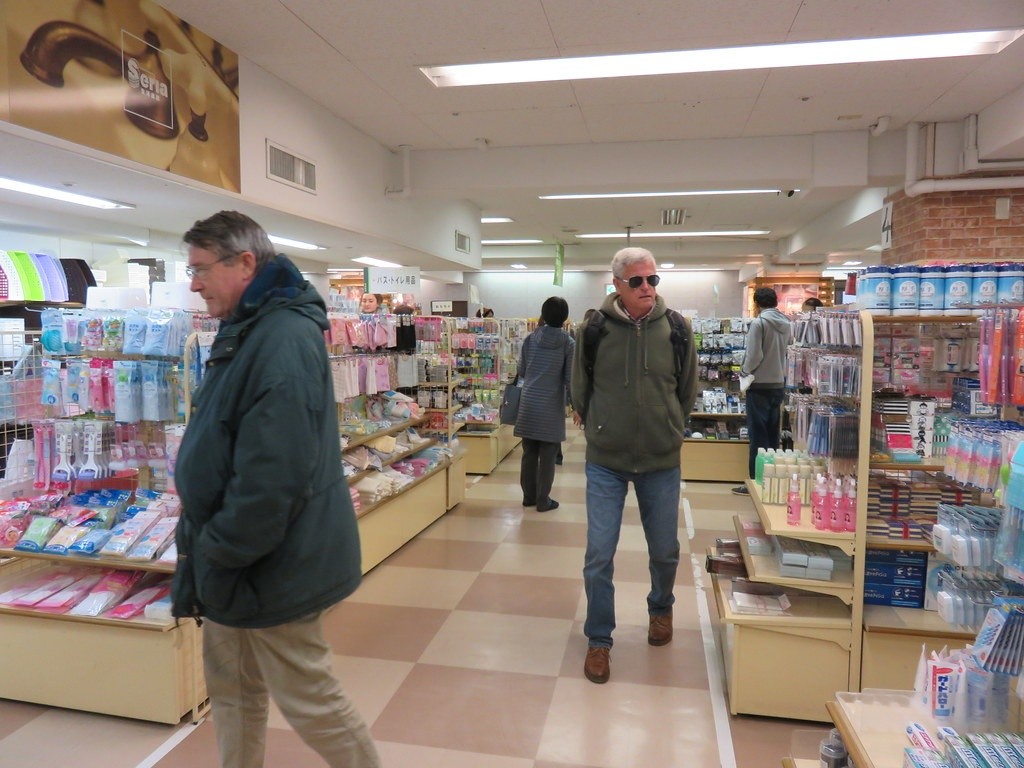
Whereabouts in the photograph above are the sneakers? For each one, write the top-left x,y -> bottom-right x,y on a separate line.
731,486 -> 750,495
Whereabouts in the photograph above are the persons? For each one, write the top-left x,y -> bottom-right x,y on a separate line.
362,293 -> 383,314
393,305 -> 414,315
569,247 -> 698,684
173,210 -> 380,768
786,505 -> 850,524
513,297 -> 575,515
476,308 -> 494,318
730,288 -> 824,496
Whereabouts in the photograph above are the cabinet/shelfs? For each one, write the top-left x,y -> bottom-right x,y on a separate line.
782,689 -> 1011,768
0,299 -> 468,725
680,308 -> 981,726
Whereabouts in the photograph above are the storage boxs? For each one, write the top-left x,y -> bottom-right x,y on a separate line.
799,540 -> 834,571
903,727 -> 1024,768
866,549 -> 928,566
770,534 -> 808,567
864,583 -> 923,608
924,552 -> 962,610
865,562 -> 924,586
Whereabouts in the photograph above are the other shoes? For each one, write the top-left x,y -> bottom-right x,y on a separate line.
536,499 -> 559,512
648,610 -> 673,646
522,500 -> 536,506
584,646 -> 611,683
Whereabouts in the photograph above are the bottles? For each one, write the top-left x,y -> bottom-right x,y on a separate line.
819,728 -> 857,768
856,263 -> 1024,314
756,448 -> 824,506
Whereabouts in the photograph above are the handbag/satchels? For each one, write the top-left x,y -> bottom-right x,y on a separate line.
500,374 -> 522,425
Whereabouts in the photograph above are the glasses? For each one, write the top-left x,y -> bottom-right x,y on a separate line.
617,275 -> 660,289
186,250 -> 246,280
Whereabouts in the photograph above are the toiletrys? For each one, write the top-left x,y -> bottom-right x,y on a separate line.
901,721 -> 1024,768
755,447 -> 857,533
